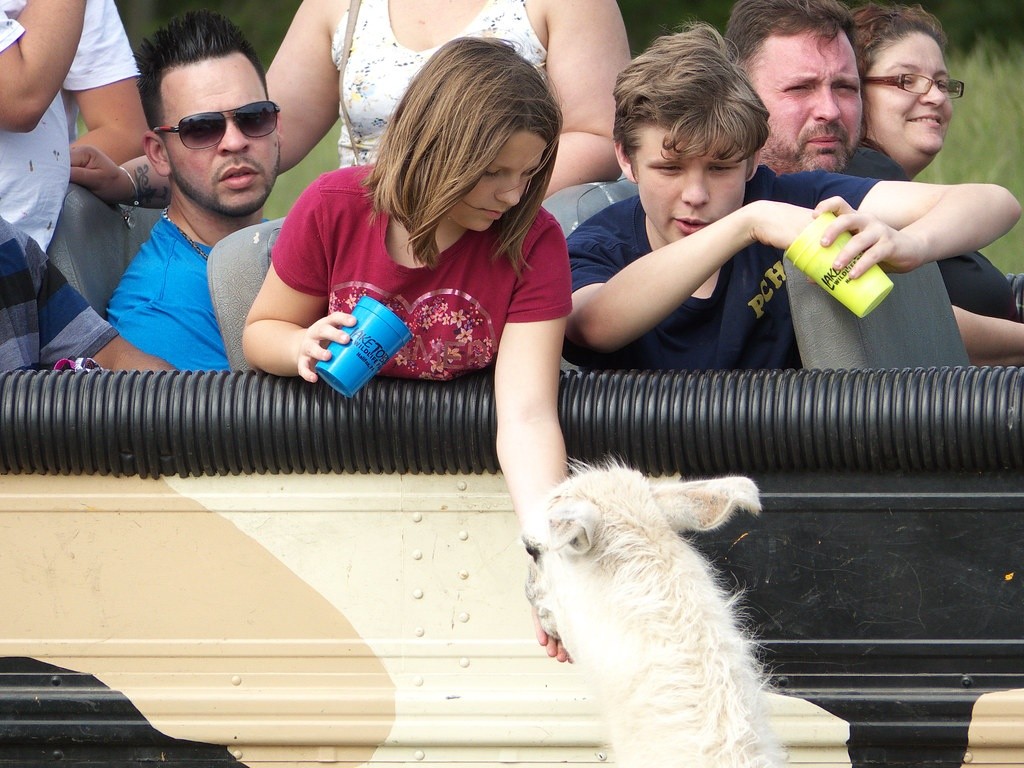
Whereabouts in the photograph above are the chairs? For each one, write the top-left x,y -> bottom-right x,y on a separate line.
48,179 -> 970,367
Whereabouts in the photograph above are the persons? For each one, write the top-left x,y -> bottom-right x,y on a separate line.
1,215 -> 178,374
69,0 -> 632,206
106,12 -> 282,374
562,24 -> 1021,373
1,1 -> 154,254
849,3 -> 1024,325
721,0 -> 865,175
245,36 -> 574,663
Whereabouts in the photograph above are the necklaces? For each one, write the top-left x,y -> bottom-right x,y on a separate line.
162,203 -> 208,262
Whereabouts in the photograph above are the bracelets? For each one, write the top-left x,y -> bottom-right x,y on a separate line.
117,166 -> 140,230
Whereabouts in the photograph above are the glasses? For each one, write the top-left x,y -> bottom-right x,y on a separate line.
153,100 -> 281,150
858,72 -> 964,99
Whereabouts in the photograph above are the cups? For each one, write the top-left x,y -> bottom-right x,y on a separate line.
784,212 -> 895,319
314,295 -> 414,399
